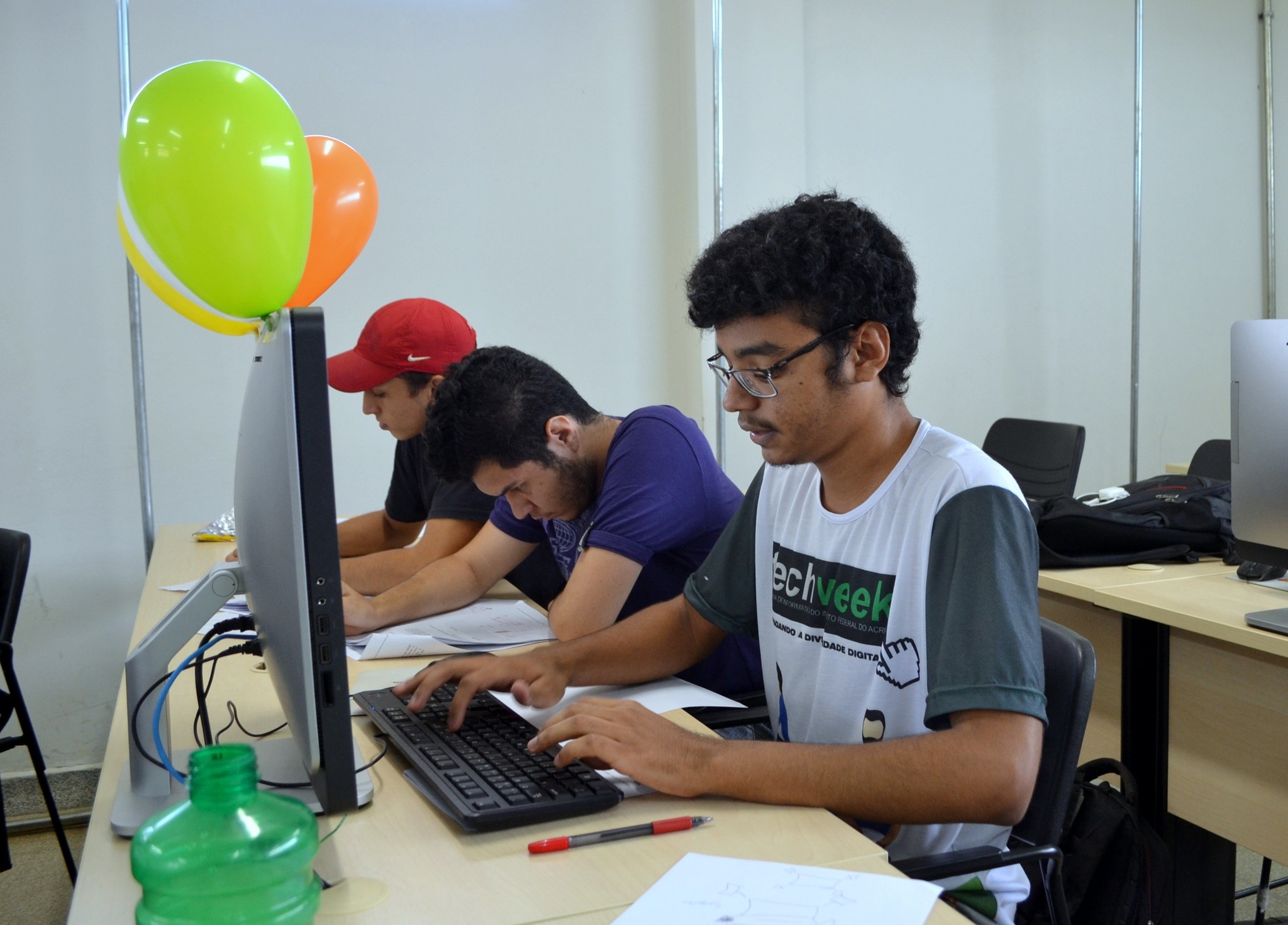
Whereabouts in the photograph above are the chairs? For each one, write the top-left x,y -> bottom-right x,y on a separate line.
1184,435 -> 1231,482
0,526 -> 76,886
984,419 -> 1084,510
891,613 -> 1094,924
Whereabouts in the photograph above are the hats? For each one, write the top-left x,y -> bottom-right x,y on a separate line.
325,298 -> 477,393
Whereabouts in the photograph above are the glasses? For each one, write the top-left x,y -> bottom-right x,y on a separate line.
706,320 -> 866,396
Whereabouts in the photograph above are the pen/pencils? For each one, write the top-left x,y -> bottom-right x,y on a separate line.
528,816 -> 714,853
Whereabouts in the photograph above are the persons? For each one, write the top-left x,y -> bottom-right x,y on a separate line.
392,190 -> 1048,925
341,345 -> 759,738
226,298 -> 564,607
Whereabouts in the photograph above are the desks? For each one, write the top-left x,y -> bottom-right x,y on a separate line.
1036,562 -> 1288,925
64,518 -> 974,923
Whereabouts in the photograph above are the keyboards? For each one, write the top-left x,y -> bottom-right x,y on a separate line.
354,685 -> 624,832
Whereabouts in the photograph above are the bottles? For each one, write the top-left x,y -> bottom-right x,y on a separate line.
130,743 -> 323,925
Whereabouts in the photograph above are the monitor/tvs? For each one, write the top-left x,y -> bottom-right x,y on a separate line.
109,305 -> 375,840
1229,319 -> 1288,636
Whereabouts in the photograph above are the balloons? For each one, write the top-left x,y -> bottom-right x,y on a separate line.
118,181 -> 264,323
120,59 -> 314,315
117,204 -> 259,336
283,135 -> 378,309
